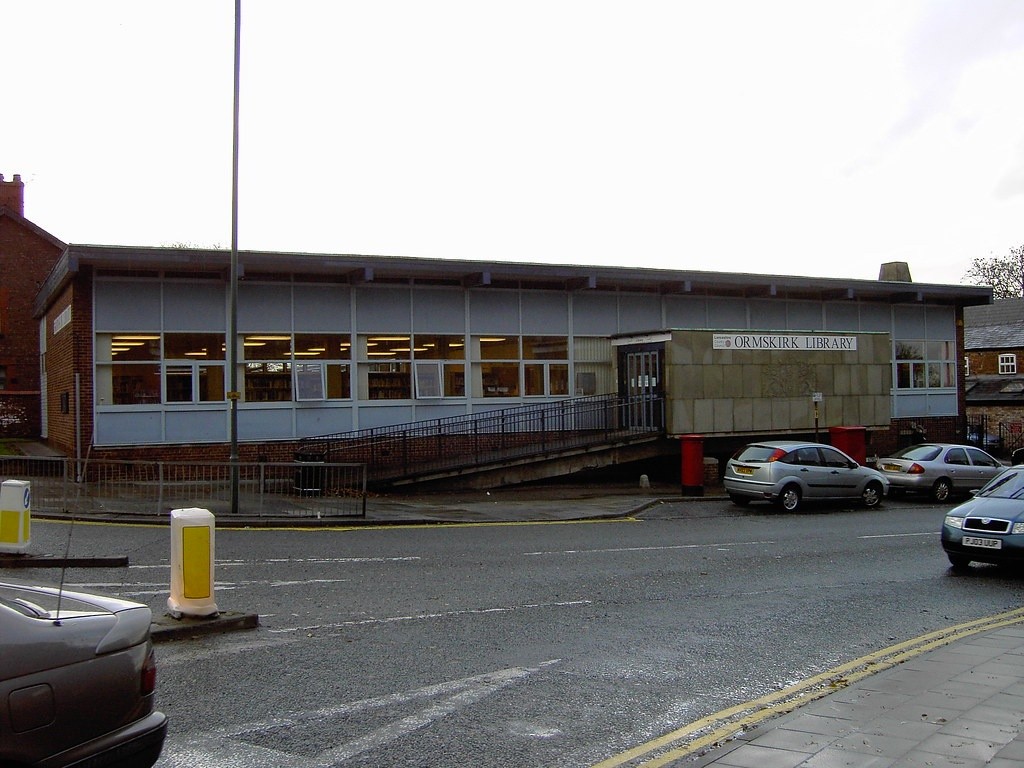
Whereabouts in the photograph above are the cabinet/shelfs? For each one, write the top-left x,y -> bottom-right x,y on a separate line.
492,366 -> 519,397
549,365 -> 567,395
450,371 -> 492,396
245,358 -> 324,402
340,359 -> 436,399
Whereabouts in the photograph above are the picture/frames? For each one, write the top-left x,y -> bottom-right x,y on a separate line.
60,391 -> 69,414
41,352 -> 47,374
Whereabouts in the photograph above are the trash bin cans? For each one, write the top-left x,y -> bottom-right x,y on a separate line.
292,451 -> 322,497
830,426 -> 866,467
680,434 -> 705,498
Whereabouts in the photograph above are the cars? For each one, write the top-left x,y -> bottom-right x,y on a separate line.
966,423 -> 1004,455
873,442 -> 1012,504
724,441 -> 891,514
0,583 -> 170,768
941,464 -> 1024,571
